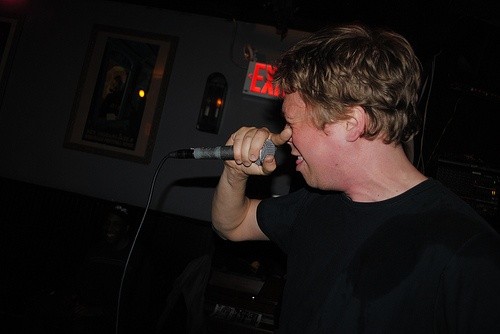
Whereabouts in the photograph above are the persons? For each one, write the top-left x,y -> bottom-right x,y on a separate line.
211,21 -> 500,334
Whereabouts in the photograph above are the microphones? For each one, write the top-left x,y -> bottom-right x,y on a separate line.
168,140 -> 276,165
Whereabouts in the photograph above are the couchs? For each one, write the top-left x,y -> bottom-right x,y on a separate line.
0,174 -> 235,334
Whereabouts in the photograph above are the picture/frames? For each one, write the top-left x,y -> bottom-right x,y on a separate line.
62,25 -> 179,165
